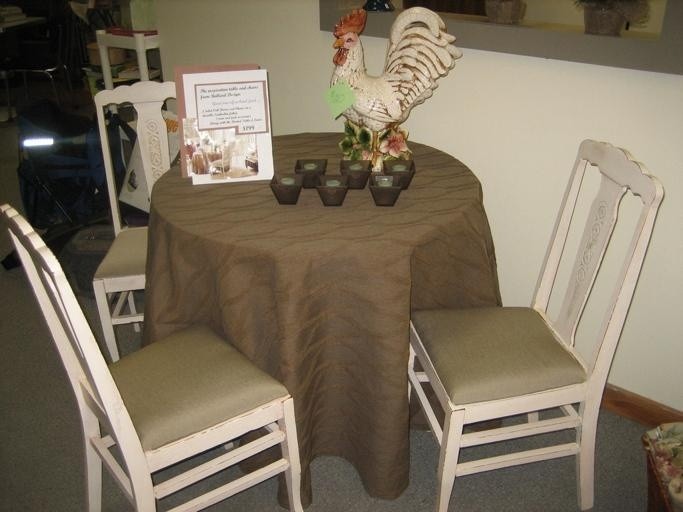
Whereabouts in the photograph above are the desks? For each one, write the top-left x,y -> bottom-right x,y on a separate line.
144,131 -> 503,510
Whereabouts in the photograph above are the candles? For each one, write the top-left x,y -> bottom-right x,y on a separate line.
349,162 -> 364,170
303,163 -> 318,170
281,177 -> 296,185
377,180 -> 393,186
391,164 -> 407,171
326,179 -> 341,187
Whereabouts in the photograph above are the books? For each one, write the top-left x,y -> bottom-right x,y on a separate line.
118,65 -> 159,79
0,6 -> 28,28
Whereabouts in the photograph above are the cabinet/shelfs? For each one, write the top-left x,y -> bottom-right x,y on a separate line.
94,25 -> 160,172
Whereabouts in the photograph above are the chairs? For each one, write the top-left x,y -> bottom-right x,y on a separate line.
91,82 -> 184,363
405,137 -> 663,509
0,20 -> 73,124
1,204 -> 305,509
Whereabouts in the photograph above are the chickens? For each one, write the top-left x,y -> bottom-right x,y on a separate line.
329,5 -> 463,166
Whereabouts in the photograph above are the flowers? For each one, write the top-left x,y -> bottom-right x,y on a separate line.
338,119 -> 413,173
